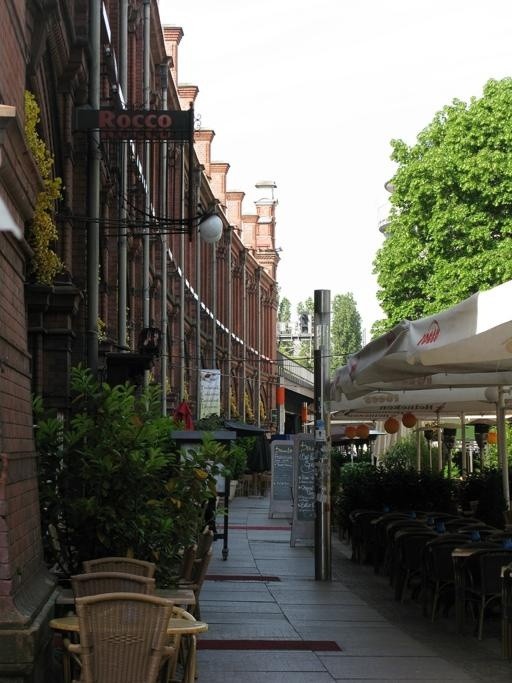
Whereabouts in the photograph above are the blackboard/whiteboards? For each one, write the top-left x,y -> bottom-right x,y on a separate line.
270,437 -> 327,538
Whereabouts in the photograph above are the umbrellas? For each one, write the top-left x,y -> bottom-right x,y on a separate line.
174,397 -> 195,431
245,432 -> 269,472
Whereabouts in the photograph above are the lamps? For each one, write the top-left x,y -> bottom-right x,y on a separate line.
402,413 -> 417,428
384,417 -> 400,434
276,386 -> 285,405
484,387 -> 500,403
355,424 -> 369,439
345,426 -> 357,440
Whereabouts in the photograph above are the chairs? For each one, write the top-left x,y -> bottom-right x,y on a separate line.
235,468 -> 273,496
348,506 -> 512,642
48,523 -> 214,683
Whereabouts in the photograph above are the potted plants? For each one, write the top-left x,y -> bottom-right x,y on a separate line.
228,445 -> 248,501
